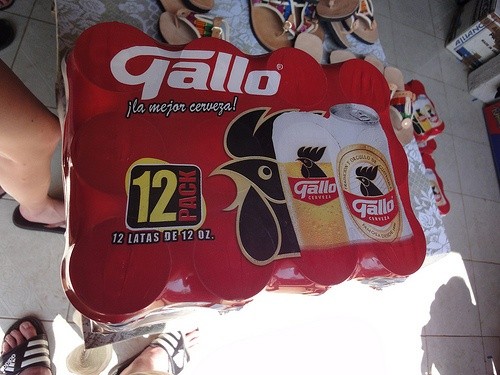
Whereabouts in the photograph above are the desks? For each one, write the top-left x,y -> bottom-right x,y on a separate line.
46,0 -> 449,351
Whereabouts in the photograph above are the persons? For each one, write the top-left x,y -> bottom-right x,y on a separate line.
0,314 -> 200,375
1,54 -> 66,236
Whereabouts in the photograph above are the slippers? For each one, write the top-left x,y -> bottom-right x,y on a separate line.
1,317 -> 52,375
251,0 -> 379,52
13,202 -> 67,234
160,0 -> 230,46
365,56 -> 414,149
107,329 -> 203,375
